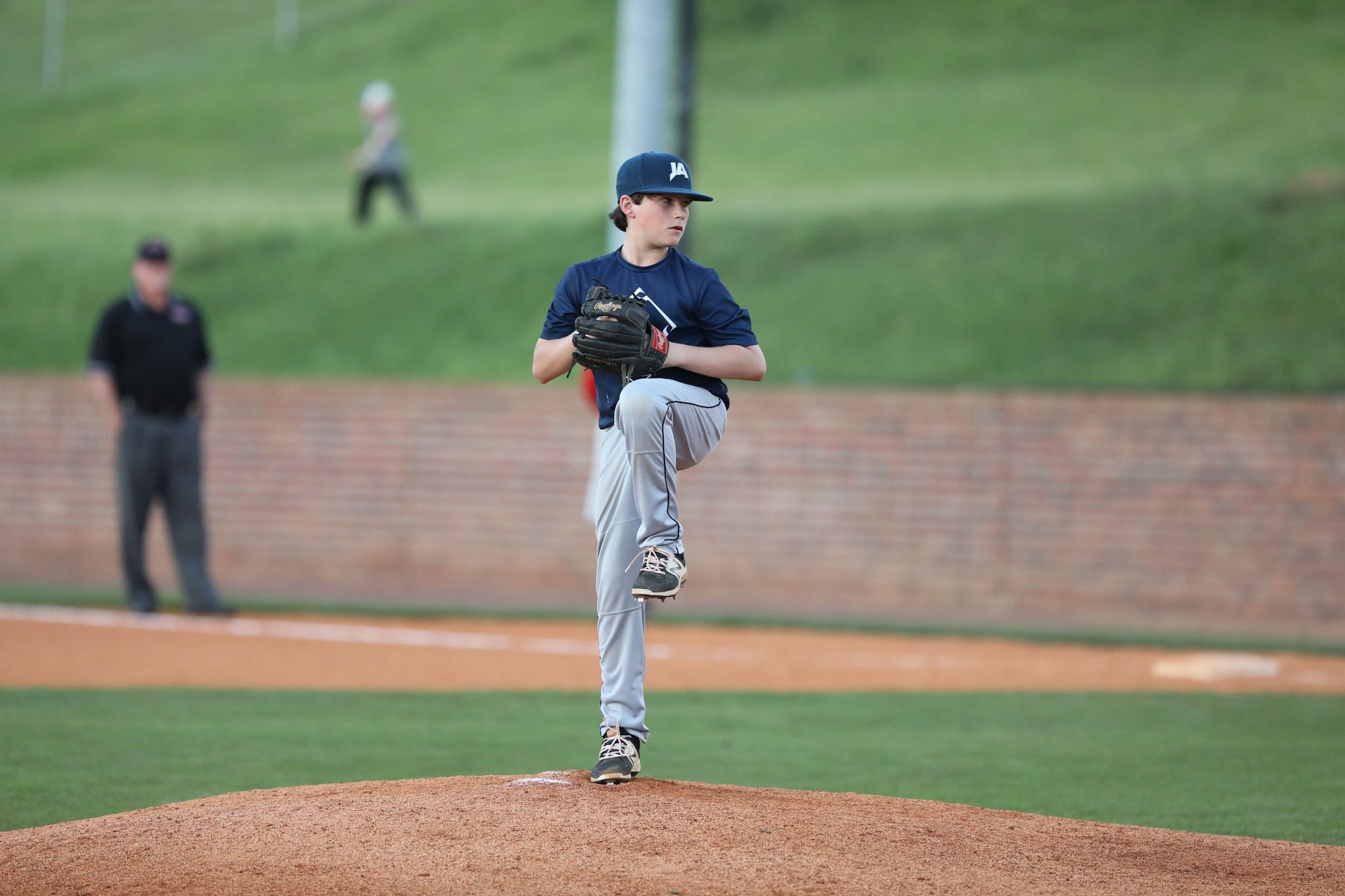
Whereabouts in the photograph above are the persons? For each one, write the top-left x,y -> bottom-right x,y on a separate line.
80,238 -> 241,615
531,148 -> 766,784
351,82 -> 418,219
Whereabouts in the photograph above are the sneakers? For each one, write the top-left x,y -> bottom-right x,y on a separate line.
589,713 -> 640,786
624,543 -> 688,601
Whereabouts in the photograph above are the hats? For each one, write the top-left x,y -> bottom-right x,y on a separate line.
142,244 -> 165,258
615,152 -> 713,203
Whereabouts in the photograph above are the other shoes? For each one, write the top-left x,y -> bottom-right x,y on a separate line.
189,601 -> 237,618
131,590 -> 157,612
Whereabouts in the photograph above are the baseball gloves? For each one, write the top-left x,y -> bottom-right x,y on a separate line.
570,284 -> 670,376
